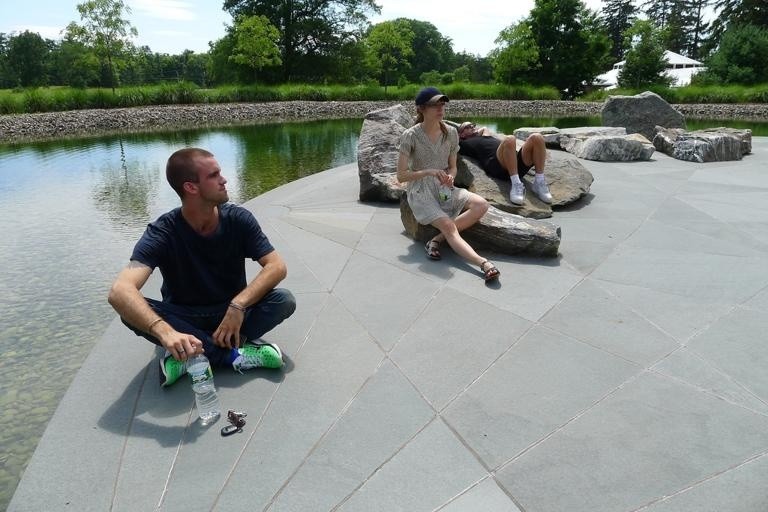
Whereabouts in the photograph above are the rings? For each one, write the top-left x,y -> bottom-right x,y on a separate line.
177,349 -> 185,353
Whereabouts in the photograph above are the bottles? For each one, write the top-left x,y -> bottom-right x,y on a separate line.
438,183 -> 453,217
187,347 -> 220,423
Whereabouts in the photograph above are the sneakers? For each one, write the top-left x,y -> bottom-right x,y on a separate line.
158,346 -> 190,390
510,182 -> 526,205
532,180 -> 553,204
232,340 -> 284,376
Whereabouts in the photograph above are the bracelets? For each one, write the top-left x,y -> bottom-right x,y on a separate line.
148,317 -> 163,332
228,301 -> 246,313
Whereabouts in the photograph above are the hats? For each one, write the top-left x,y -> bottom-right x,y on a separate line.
415,87 -> 449,105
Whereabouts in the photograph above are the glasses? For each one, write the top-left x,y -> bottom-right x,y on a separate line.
460,123 -> 476,135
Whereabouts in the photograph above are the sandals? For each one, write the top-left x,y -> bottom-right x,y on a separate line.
424,240 -> 442,260
481,260 -> 500,280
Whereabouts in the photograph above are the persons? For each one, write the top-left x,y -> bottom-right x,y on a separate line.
457,121 -> 553,204
397,86 -> 501,282
107,149 -> 296,387
